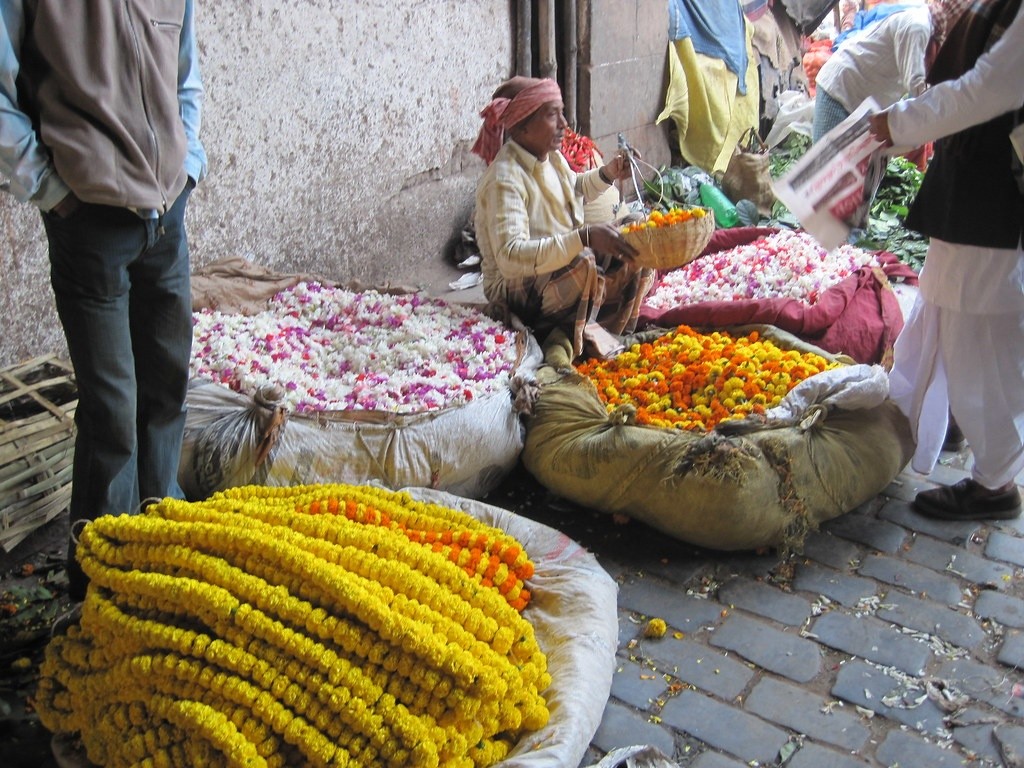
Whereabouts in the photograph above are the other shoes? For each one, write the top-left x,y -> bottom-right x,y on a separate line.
915,478 -> 1021,520
942,415 -> 968,452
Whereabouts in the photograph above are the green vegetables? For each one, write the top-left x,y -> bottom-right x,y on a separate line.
857,157 -> 928,271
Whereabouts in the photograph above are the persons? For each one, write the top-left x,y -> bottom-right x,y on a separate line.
0,0 -> 207,604
470,75 -> 654,356
840,0 -> 858,32
868,0 -> 1024,519
813,0 -> 972,255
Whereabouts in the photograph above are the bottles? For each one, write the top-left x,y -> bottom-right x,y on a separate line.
697,180 -> 739,227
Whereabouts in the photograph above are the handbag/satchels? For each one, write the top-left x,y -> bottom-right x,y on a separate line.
722,127 -> 773,220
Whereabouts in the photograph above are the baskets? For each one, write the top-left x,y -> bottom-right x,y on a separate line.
619,208 -> 715,268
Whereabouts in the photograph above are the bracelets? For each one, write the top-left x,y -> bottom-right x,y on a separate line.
586,225 -> 590,246
